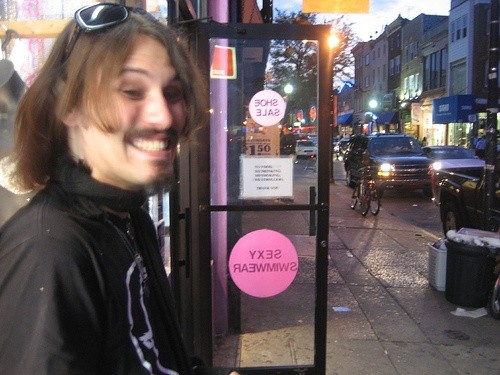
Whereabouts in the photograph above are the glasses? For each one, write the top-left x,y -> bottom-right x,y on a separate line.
60,2 -> 161,64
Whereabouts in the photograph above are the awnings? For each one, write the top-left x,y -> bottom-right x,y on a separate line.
374,112 -> 400,124
336,112 -> 353,126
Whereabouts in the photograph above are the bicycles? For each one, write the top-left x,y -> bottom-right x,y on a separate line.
350,165 -> 381,216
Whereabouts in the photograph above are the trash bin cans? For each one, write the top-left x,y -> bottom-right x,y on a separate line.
426,241 -> 446,293
445,231 -> 499,309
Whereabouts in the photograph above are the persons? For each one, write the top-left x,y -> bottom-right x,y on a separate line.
0,3 -> 208,375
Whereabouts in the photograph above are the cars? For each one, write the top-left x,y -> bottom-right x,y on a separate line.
332,136 -> 352,158
295,139 -> 318,158
423,144 -> 486,170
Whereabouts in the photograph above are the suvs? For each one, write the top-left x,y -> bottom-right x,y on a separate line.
344,132 -> 431,199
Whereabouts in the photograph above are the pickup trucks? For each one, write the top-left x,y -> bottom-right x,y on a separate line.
432,166 -> 500,238
